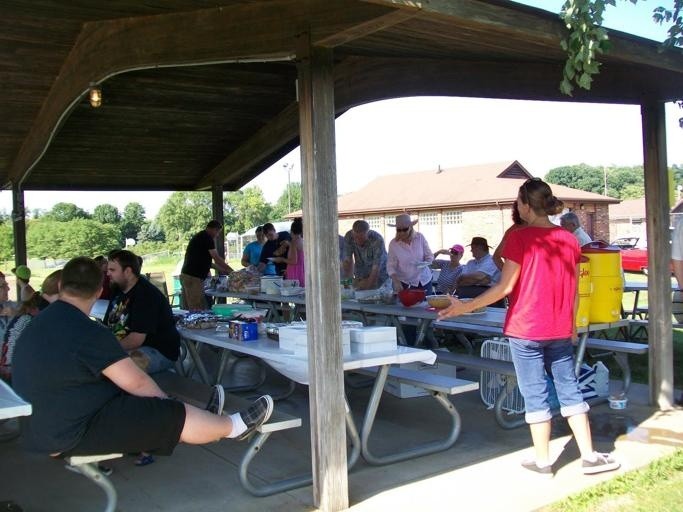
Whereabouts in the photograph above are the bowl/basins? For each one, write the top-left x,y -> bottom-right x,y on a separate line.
380,292 -> 399,305
426,294 -> 459,309
265,325 -> 278,340
398,290 -> 425,307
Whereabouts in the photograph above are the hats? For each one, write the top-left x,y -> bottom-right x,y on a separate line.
11,265 -> 31,280
466,237 -> 494,250
450,244 -> 464,253
387,214 -> 419,227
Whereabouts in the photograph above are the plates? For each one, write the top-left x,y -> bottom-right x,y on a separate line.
267,257 -> 275,261
410,262 -> 432,269
462,311 -> 489,316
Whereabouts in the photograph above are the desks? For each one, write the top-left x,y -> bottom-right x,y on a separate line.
0,374 -> 34,444
623,281 -> 683,344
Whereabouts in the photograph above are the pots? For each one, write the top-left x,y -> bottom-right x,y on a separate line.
259,275 -> 284,293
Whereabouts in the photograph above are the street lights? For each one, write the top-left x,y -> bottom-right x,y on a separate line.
282,163 -> 293,213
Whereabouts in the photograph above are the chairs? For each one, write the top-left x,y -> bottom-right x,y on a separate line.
145,271 -> 183,310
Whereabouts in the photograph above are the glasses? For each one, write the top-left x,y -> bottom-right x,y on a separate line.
397,226 -> 410,232
450,250 -> 459,255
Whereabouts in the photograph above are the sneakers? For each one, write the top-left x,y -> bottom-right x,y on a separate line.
64,463 -> 113,475
228,394 -> 274,441
206,384 -> 225,442
581,451 -> 621,474
520,461 -> 554,478
134,452 -> 156,466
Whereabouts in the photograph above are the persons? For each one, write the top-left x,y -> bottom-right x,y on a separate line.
456,236 -> 502,285
493,200 -> 529,273
669,217 -> 683,293
426,245 -> 464,297
105,248 -> 181,374
11,255 -> 274,459
240,216 -> 388,290
560,213 -> 593,248
435,177 -> 622,479
179,219 -> 234,310
0,269 -> 64,386
0,271 -> 21,360
385,214 -> 435,350
11,265 -> 37,301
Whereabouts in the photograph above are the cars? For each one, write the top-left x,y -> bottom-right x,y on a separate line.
612,238 -> 649,278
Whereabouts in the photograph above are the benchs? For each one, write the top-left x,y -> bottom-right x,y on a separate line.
62,451 -> 126,468
154,377 -> 303,435
252,302 -> 648,356
630,319 -> 683,329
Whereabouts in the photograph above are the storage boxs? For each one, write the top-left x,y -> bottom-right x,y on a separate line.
384,362 -> 457,399
